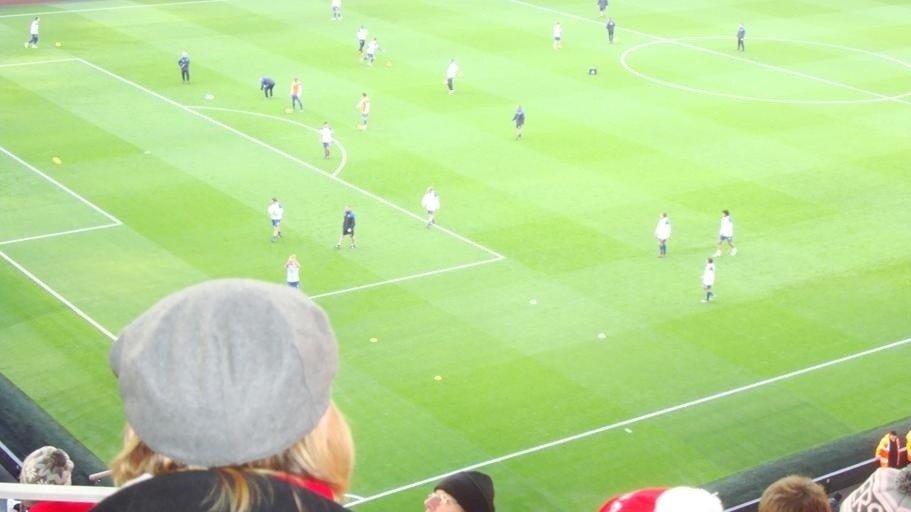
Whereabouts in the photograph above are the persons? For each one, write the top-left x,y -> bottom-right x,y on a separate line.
82,277 -> 353,512
421,187 -> 439,224
446,59 -> 456,90
423,470 -> 495,512
24,16 -> 40,48
178,54 -> 190,83
357,26 -> 380,63
332,1 -> 342,21
261,76 -> 371,158
657,210 -> 735,302
266,197 -> 356,288
736,25 -> 745,51
552,1 -> 616,49
512,107 -> 524,139
5,446 -> 75,512
599,430 -> 911,512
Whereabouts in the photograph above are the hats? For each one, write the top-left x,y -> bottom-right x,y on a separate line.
840,466 -> 911,512
434,470 -> 495,512
109,278 -> 338,468
600,484 -> 724,512
20,446 -> 75,485
